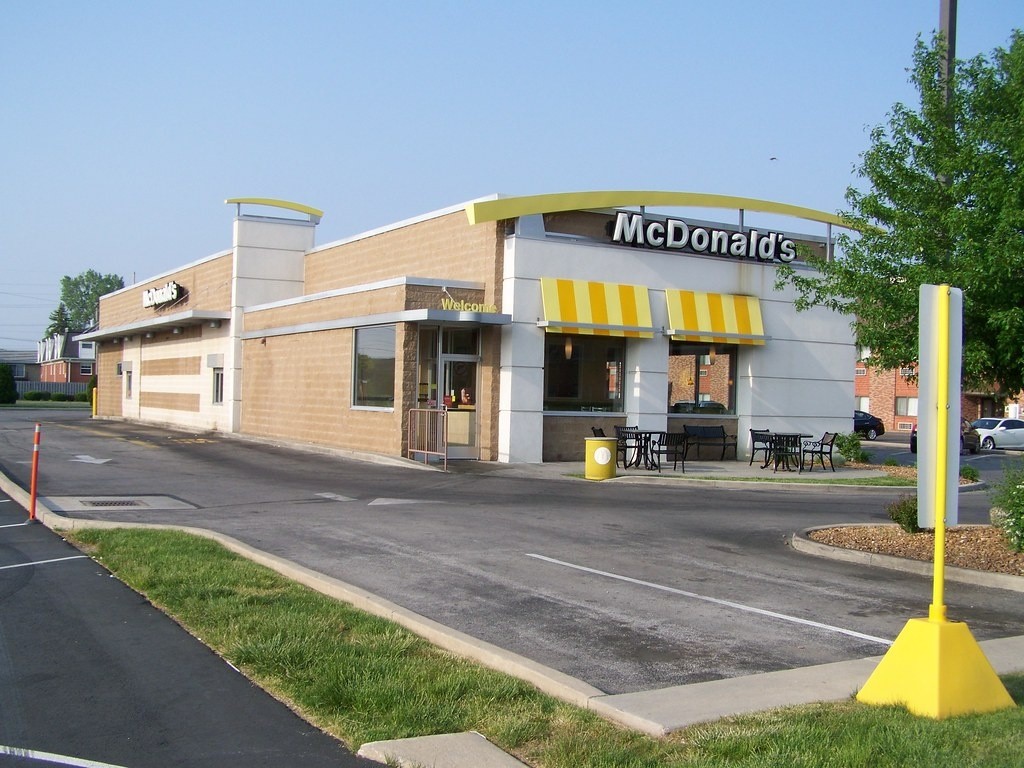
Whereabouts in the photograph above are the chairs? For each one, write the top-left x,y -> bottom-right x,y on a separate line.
591,425 -> 687,473
750,429 -> 838,474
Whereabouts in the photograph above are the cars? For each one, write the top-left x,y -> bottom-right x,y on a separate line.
909,415 -> 981,454
854,410 -> 886,440
972,418 -> 1024,452
673,400 -> 728,414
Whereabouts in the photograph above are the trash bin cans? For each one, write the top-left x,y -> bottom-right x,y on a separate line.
583,437 -> 619,481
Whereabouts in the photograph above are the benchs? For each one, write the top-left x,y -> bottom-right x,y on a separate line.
682,425 -> 737,461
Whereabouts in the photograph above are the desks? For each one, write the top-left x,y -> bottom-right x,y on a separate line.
755,432 -> 813,470
621,430 -> 666,469
